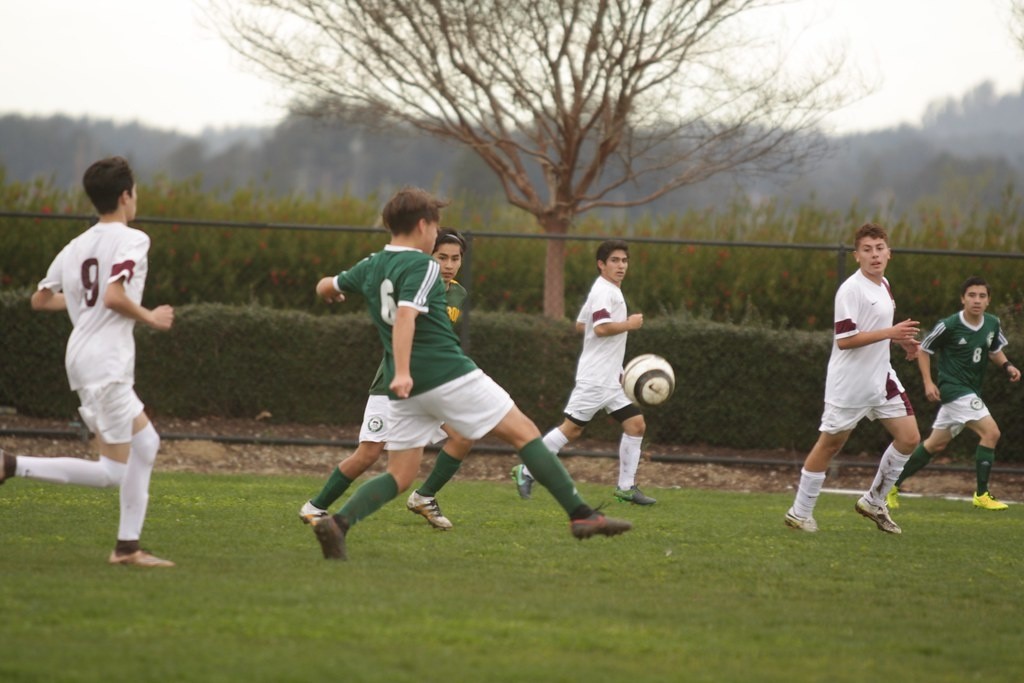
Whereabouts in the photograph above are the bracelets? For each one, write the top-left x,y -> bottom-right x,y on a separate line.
1003,361 -> 1013,370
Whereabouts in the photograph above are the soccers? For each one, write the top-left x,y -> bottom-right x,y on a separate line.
622,350 -> 676,407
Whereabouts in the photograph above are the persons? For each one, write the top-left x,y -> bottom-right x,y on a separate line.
885,276 -> 1021,512
510,241 -> 658,506
0,158 -> 174,569
785,222 -> 921,533
312,186 -> 636,562
300,227 -> 476,530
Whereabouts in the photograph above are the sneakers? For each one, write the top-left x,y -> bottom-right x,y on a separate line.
571,502 -> 632,541
510,464 -> 534,499
614,483 -> 656,505
406,489 -> 453,530
109,547 -> 176,567
784,506 -> 820,532
855,496 -> 901,534
0,448 -> 4,484
886,485 -> 900,509
299,499 -> 329,527
973,491 -> 1009,510
313,516 -> 347,560
